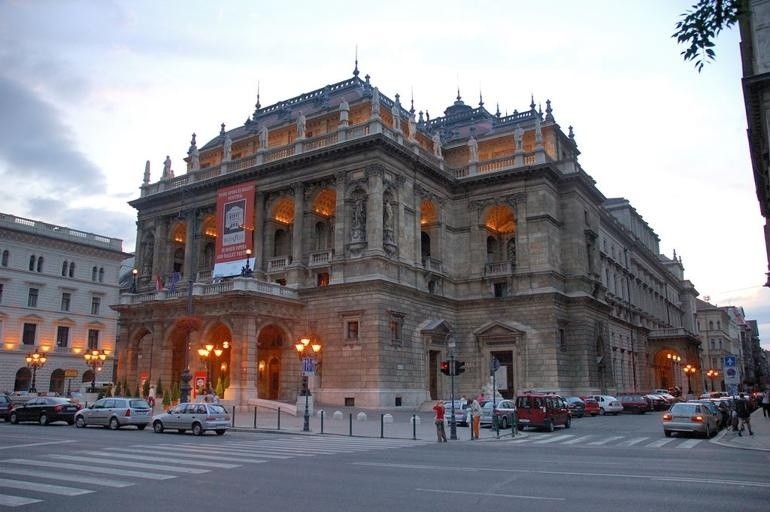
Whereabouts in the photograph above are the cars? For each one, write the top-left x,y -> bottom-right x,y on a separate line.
564,388 -> 679,418
152,401 -> 233,435
9,390 -> 80,404
9,395 -> 80,425
480,400 -> 515,430
0,394 -> 12,421
660,392 -> 765,439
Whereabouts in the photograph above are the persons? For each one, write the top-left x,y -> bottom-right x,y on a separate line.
514,123 -> 524,150
460,395 -> 466,411
296,111 -> 307,137
735,394 -> 753,436
205,386 -> 219,403
761,389 -> 769,418
478,394 -> 485,404
191,145 -> 200,170
224,135 -> 232,160
149,386 -> 155,407
468,135 -> 479,161
432,131 -> 442,158
257,122 -> 267,149
408,113 -> 417,141
391,101 -> 401,128
466,397 -> 482,439
67,388 -> 71,396
339,98 -> 350,126
164,154 -> 171,176
432,400 -> 447,443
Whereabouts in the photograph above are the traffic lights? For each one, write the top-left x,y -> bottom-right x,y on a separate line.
440,360 -> 450,376
455,360 -> 465,375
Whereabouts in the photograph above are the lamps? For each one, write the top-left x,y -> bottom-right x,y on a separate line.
129,268 -> 139,293
241,249 -> 254,277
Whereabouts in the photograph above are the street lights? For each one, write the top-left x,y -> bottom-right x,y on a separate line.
244,249 -> 252,276
707,368 -> 718,392
197,340 -> 223,394
84,347 -> 111,392
295,335 -> 323,431
684,364 -> 696,394
25,347 -> 47,393
132,268 -> 139,293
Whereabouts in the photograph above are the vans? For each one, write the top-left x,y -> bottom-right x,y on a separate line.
74,395 -> 154,431
514,390 -> 573,432
442,400 -> 468,423
